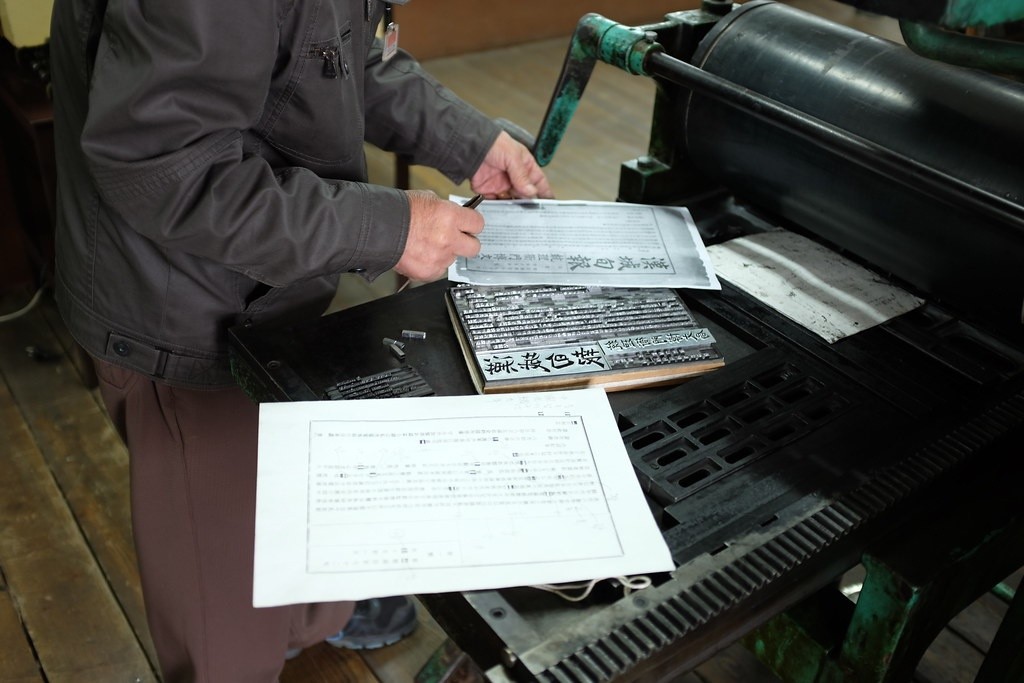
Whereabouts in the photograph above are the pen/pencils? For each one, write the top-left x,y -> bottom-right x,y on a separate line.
397,193 -> 485,292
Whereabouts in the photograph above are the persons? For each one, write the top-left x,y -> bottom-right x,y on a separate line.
53,0 -> 555,683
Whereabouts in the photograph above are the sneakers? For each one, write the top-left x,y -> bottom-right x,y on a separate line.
326,593 -> 419,650
